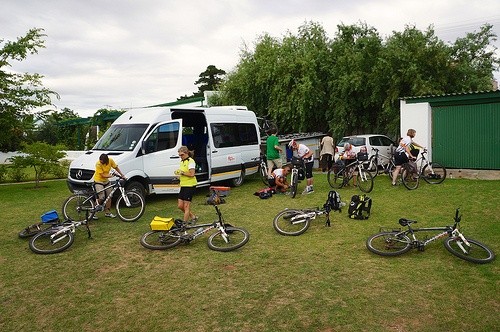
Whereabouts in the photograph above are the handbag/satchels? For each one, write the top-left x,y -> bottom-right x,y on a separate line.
40,210 -> 59,223
150,215 -> 174,230
291,156 -> 304,166
357,152 -> 369,162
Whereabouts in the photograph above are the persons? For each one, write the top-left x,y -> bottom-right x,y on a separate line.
93,154 -> 126,219
320,131 -> 335,172
339,142 -> 358,187
288,139 -> 314,195
267,128 -> 283,178
268,164 -> 292,194
174,146 -> 198,225
392,129 -> 426,187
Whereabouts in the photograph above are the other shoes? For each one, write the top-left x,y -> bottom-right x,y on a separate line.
191,216 -> 198,225
281,188 -> 286,192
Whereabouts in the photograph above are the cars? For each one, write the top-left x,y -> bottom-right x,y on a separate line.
334,133 -> 399,171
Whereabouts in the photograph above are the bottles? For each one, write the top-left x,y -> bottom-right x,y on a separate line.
192,227 -> 204,235
449,172 -> 452,179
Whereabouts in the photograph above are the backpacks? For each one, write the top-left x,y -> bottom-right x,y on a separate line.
328,190 -> 341,210
348,194 -> 372,220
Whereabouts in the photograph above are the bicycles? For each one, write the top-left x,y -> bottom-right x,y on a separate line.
327,143 -> 397,194
272,197 -> 334,236
401,150 -> 447,190
139,205 -> 250,252
251,153 -> 277,186
18,209 -> 92,255
255,112 -> 278,137
61,173 -> 146,222
366,207 -> 495,264
289,156 -> 304,198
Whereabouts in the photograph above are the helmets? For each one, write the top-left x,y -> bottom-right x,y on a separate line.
288,139 -> 295,149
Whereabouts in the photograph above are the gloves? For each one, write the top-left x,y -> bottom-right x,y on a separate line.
339,155 -> 343,160
174,170 -> 182,175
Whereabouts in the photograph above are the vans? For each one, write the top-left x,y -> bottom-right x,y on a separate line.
66,104 -> 263,208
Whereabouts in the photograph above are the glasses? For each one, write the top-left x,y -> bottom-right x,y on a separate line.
179,154 -> 184,156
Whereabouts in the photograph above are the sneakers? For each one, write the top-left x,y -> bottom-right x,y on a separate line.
105,213 -> 116,218
93,215 -> 99,219
302,189 -> 314,194
